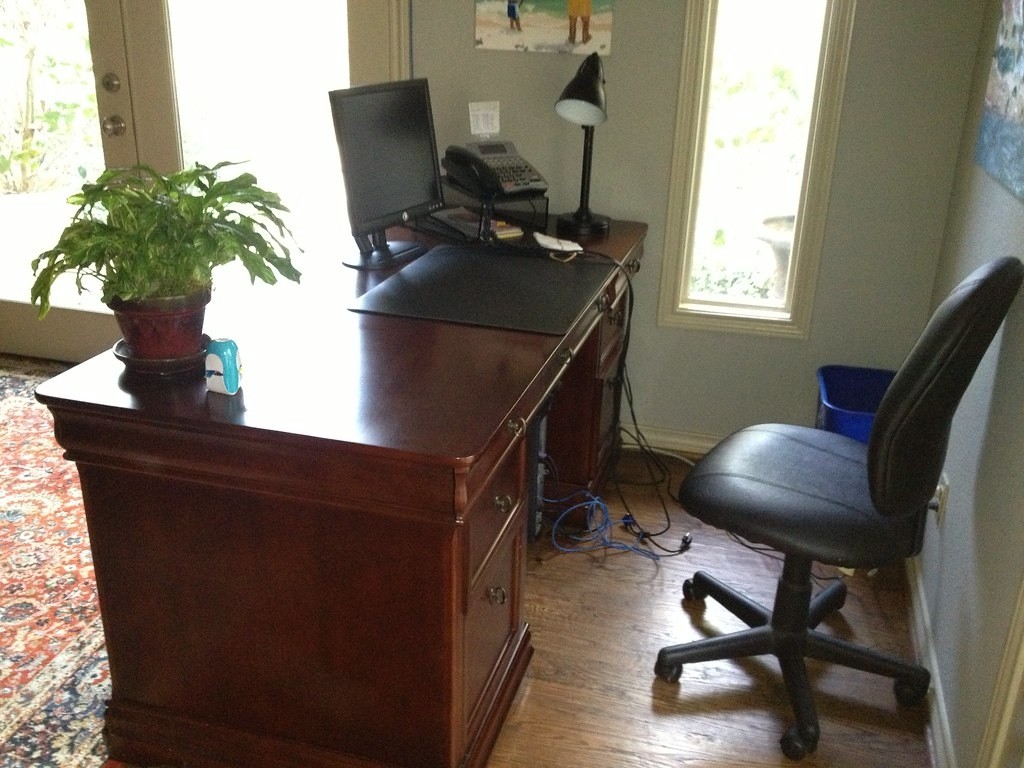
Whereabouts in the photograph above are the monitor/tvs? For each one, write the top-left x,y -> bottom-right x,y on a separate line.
329,77 -> 447,270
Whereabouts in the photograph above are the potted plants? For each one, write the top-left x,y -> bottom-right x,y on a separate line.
29,159 -> 308,361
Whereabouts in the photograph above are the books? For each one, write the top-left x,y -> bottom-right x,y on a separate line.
430,207 -> 524,240
480,232 -> 524,241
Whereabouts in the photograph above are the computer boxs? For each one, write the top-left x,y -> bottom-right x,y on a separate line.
526,409 -> 547,542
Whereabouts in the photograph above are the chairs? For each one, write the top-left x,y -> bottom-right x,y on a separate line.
662,256 -> 1024,761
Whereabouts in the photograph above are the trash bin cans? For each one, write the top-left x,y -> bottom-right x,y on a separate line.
815,364 -> 900,446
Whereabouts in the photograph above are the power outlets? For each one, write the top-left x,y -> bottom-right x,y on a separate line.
934,472 -> 950,527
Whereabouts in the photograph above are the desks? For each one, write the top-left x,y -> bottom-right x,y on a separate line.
33,223 -> 650,768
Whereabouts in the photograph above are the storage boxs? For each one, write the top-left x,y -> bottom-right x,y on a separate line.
815,363 -> 897,442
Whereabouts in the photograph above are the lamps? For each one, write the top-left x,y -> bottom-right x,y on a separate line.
554,51 -> 613,236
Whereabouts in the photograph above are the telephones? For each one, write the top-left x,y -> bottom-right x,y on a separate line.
438,137 -> 550,202
